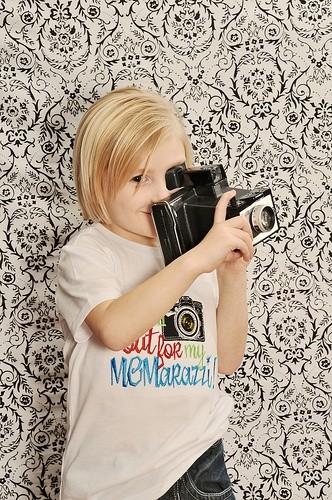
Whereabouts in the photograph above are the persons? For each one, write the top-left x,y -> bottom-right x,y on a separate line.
54,87 -> 257,500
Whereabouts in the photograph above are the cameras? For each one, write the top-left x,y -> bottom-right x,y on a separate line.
152,163 -> 279,267
